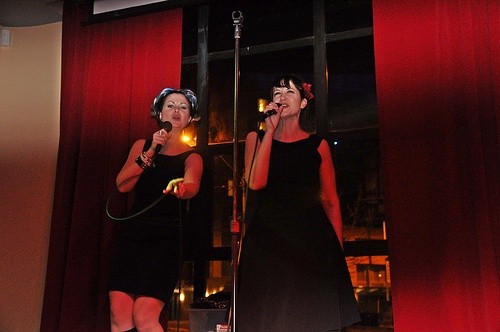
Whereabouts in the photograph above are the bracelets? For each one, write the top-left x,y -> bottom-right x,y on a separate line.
140,152 -> 152,167
135,156 -> 148,170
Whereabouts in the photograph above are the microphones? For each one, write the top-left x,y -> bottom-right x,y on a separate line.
155,121 -> 172,152
255,103 -> 281,121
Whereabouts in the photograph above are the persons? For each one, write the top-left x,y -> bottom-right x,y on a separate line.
107,87 -> 203,332
225,75 -> 362,332
